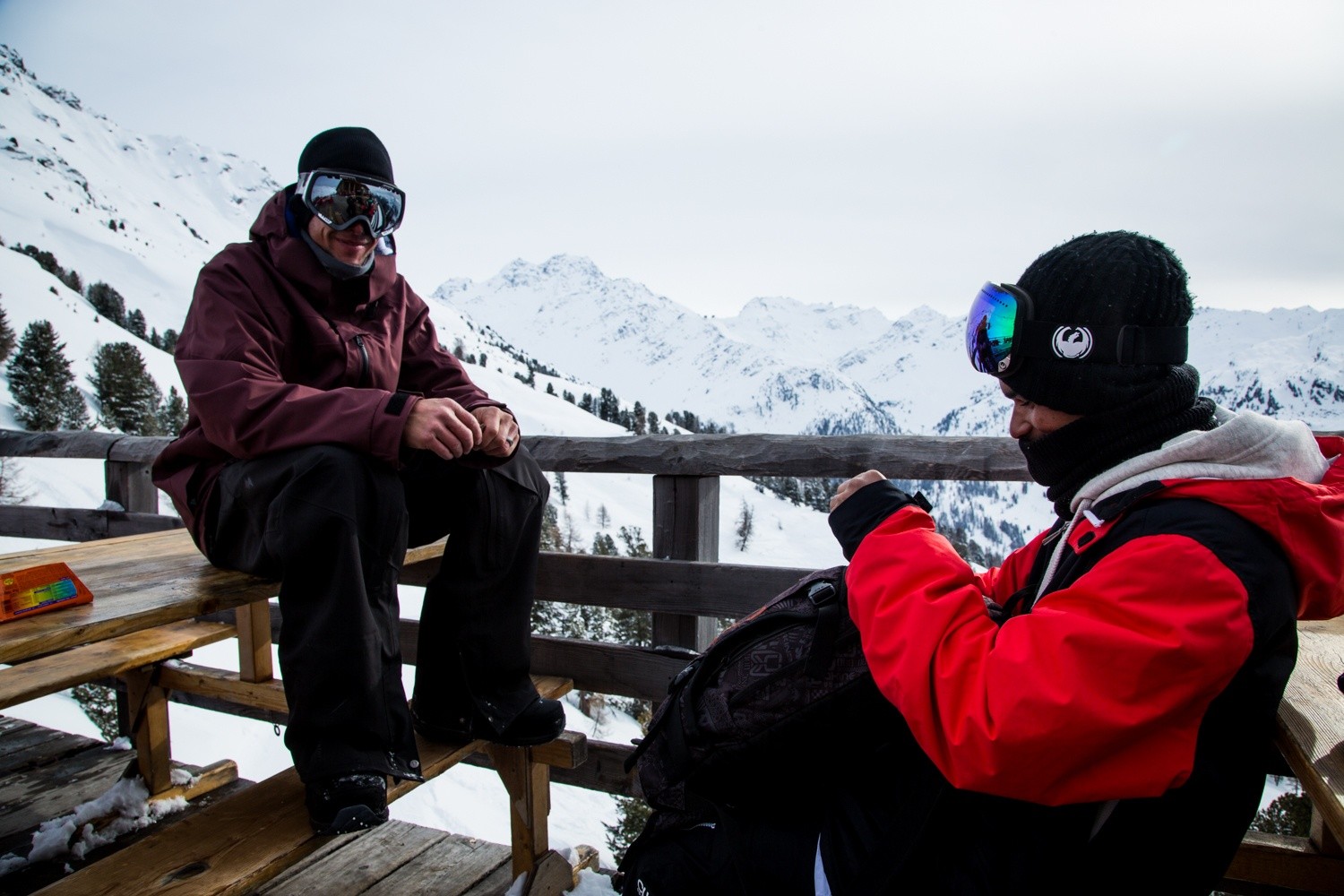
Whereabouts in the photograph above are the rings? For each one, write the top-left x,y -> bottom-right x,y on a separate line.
507,438 -> 513,446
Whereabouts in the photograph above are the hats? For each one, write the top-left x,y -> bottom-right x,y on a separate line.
998,228 -> 1191,413
297,126 -> 394,186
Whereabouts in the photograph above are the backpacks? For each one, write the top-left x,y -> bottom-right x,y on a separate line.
633,566 -> 892,844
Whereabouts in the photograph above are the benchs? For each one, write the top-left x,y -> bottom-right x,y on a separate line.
1,615 -> 588,896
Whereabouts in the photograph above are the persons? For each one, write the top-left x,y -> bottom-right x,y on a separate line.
150,128 -> 566,840
347,194 -> 375,219
826,230 -> 1344,896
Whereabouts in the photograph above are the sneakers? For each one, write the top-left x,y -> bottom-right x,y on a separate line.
409,689 -> 566,747
309,769 -> 388,832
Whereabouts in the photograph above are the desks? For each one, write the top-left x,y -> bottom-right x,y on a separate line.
0,521 -> 454,711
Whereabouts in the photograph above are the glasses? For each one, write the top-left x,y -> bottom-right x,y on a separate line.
304,170 -> 407,236
965,281 -> 1031,382
